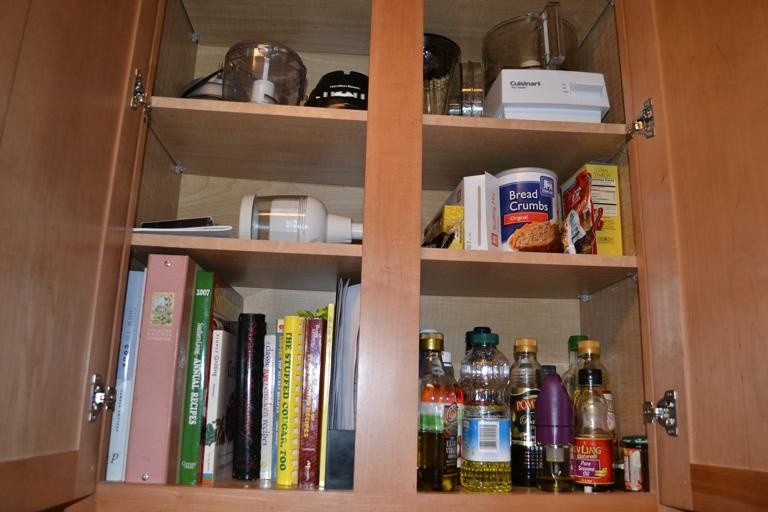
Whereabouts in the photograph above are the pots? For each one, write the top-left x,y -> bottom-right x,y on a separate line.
176,39 -> 309,106
479,1 -> 578,103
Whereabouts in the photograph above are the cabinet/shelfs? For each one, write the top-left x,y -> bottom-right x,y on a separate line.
0,2 -> 768,511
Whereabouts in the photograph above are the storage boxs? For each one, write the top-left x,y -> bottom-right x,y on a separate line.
482,67 -> 611,123
421,160 -> 625,255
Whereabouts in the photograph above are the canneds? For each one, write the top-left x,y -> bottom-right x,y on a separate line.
621,435 -> 647,492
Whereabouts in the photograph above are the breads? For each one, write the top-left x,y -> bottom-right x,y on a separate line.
511,220 -> 563,253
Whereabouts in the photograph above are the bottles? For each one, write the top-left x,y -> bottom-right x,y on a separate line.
417,325 -> 616,491
